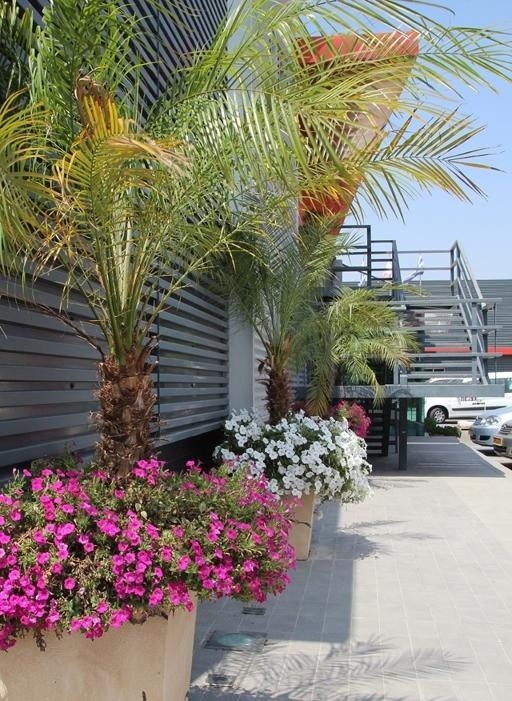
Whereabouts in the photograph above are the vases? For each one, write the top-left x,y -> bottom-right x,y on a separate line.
0,588 -> 196,701
271,472 -> 312,560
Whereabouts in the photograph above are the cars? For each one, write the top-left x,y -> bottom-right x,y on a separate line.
403,370 -> 510,459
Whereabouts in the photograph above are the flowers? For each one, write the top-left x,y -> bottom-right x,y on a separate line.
214,397 -> 372,508
0,449 -> 308,645
